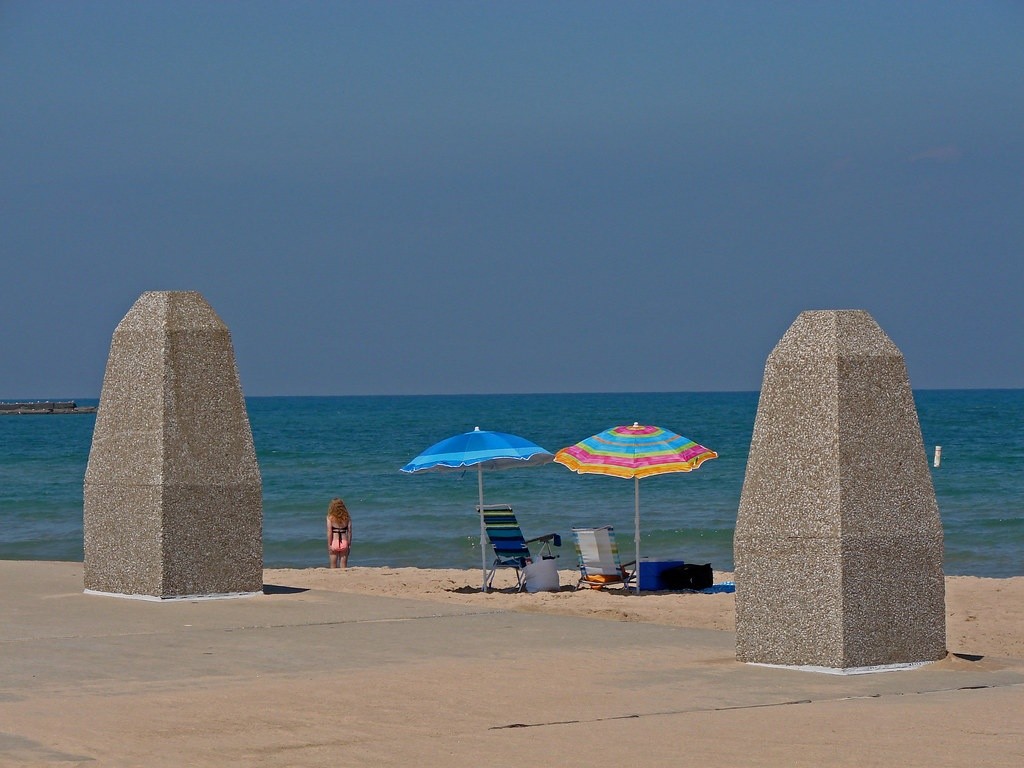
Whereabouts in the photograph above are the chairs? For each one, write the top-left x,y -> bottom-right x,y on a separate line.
475,503 -> 560,592
572,525 -> 639,593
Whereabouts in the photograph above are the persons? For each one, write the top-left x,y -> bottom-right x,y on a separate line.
325,497 -> 353,569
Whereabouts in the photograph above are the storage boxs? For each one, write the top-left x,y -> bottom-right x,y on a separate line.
639,561 -> 685,591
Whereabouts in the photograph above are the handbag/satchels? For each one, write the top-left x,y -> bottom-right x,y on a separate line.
658,563 -> 713,590
521,554 -> 560,593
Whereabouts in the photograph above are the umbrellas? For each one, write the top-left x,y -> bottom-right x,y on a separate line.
553,422 -> 718,594
398,425 -> 556,593
